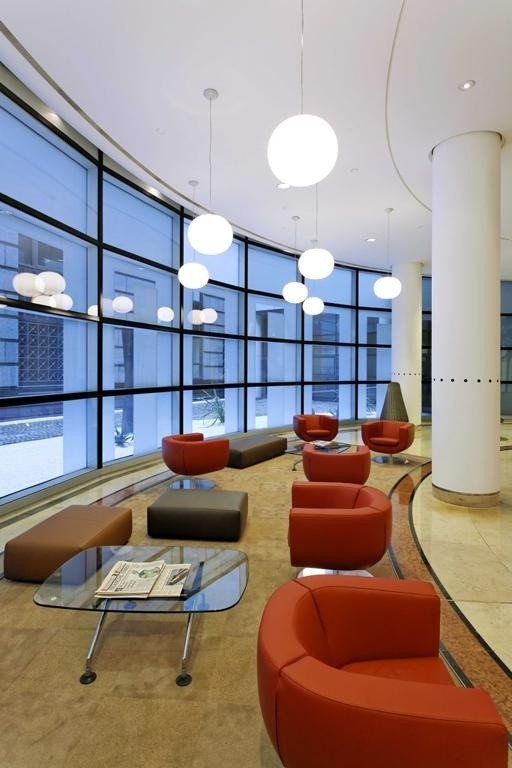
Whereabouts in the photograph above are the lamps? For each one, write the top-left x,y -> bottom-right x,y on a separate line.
297,185 -> 339,283
185,81 -> 237,258
371,207 -> 404,302
300,294 -> 326,317
177,177 -> 211,293
266,0 -> 343,190
278,215 -> 311,306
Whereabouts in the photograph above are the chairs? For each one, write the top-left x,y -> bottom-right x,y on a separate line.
287,479 -> 393,579
162,433 -> 230,490
256,576 -> 509,768
302,443 -> 371,485
293,413 -> 339,449
361,419 -> 416,465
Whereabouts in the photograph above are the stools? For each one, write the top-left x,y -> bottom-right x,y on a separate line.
4,506 -> 132,586
226,434 -> 287,468
147,490 -> 248,542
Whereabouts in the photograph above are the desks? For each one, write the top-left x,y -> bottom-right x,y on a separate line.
284,441 -> 351,471
32,545 -> 249,686
